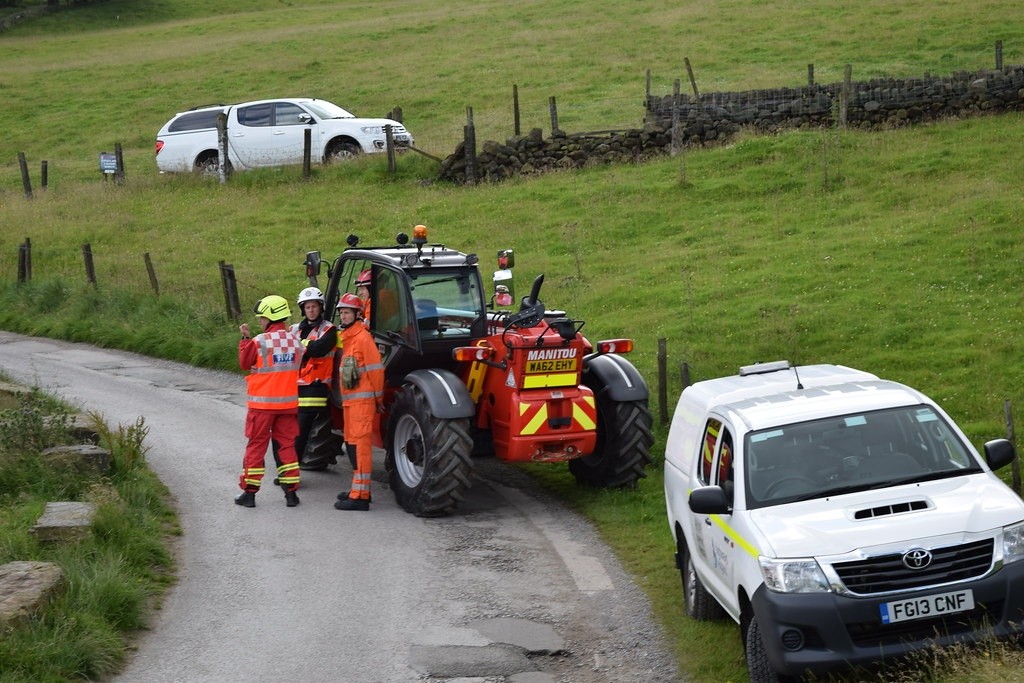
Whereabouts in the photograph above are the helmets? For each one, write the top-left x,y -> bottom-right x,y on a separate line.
254,296 -> 292,321
296,287 -> 324,304
335,293 -> 364,309
354,268 -> 372,287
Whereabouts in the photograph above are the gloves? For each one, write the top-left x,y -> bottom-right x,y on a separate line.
342,357 -> 357,389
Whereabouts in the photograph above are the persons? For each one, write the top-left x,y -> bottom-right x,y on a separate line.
232,296 -> 303,507
333,293 -> 385,511
273,288 -> 338,485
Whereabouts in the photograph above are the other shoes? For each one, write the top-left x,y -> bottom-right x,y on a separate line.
334,499 -> 370,510
286,492 -> 299,506
274,479 -> 280,485
234,493 -> 256,506
337,491 -> 372,503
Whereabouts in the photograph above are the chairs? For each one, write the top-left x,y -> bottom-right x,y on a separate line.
854,421 -> 926,479
779,425 -> 837,474
413,304 -> 439,330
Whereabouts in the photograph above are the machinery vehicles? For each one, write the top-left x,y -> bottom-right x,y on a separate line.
294,223 -> 656,517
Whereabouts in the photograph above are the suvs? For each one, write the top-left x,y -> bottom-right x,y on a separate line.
662,361 -> 1023,683
155,97 -> 414,174
